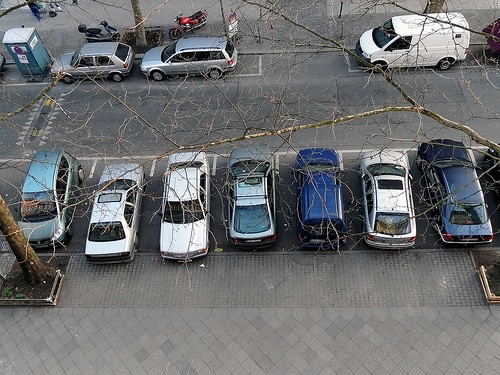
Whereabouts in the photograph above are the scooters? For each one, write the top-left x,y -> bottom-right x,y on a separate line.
78,20 -> 121,43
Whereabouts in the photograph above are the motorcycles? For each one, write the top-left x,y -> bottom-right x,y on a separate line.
169,9 -> 208,41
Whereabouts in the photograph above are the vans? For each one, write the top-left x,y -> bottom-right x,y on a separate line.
356,12 -> 470,73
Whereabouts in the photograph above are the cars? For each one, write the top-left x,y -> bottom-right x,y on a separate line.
417,139 -> 500,245
159,152 -> 211,262
18,149 -> 84,250
49,42 -> 135,84
357,150 -> 417,250
291,148 -> 348,250
141,36 -> 238,81
224,143 -> 278,250
85,163 -> 145,264
